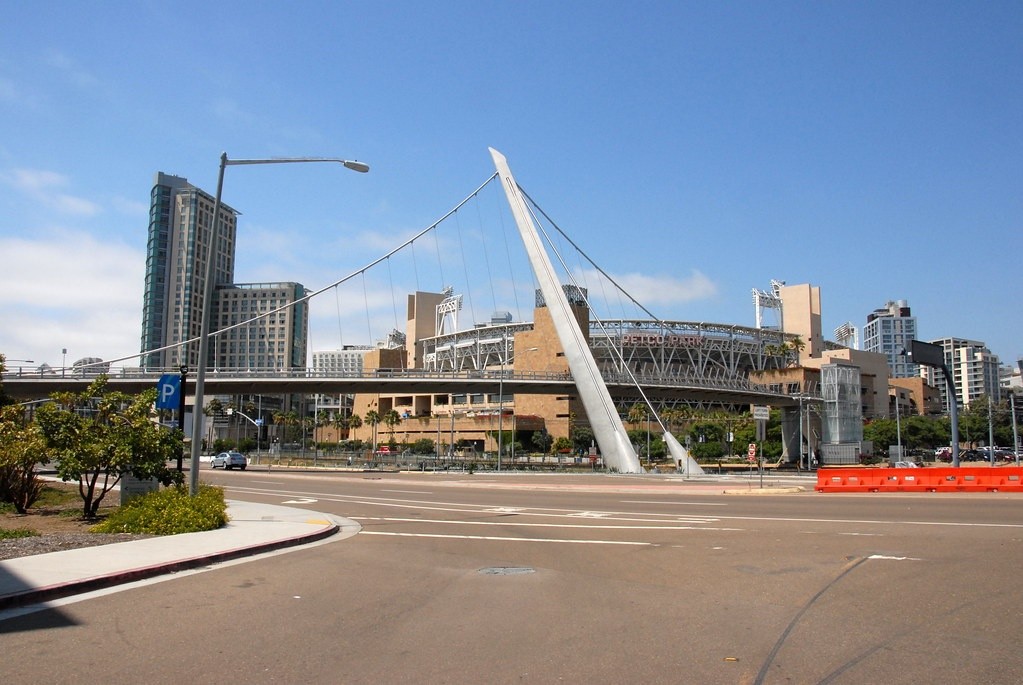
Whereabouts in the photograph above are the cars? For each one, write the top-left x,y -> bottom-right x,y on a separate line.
903,445 -> 1023,462
375,446 -> 401,456
210,453 -> 248,470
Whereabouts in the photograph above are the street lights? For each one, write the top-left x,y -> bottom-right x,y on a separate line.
62,349 -> 67,378
186,150 -> 371,500
498,347 -> 538,470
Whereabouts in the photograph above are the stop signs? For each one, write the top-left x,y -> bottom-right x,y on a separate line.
748,443 -> 756,456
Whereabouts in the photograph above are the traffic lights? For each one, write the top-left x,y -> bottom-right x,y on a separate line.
233,411 -> 236,418
